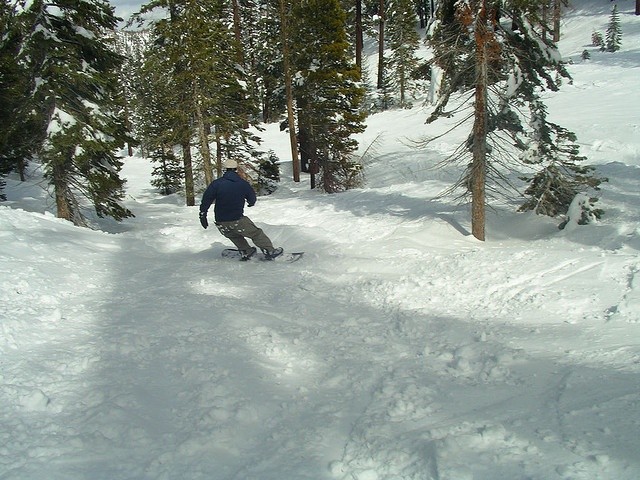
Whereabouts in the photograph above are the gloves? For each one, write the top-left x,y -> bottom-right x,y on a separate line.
247,203 -> 254,207
199,212 -> 208,229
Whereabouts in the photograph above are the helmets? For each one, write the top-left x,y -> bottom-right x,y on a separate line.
225,159 -> 238,168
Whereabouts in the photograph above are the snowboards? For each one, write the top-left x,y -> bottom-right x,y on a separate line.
222,249 -> 304,263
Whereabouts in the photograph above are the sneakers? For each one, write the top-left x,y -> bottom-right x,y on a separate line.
241,247 -> 256,259
265,247 -> 283,260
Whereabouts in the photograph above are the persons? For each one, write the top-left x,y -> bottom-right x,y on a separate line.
199,159 -> 283,261
